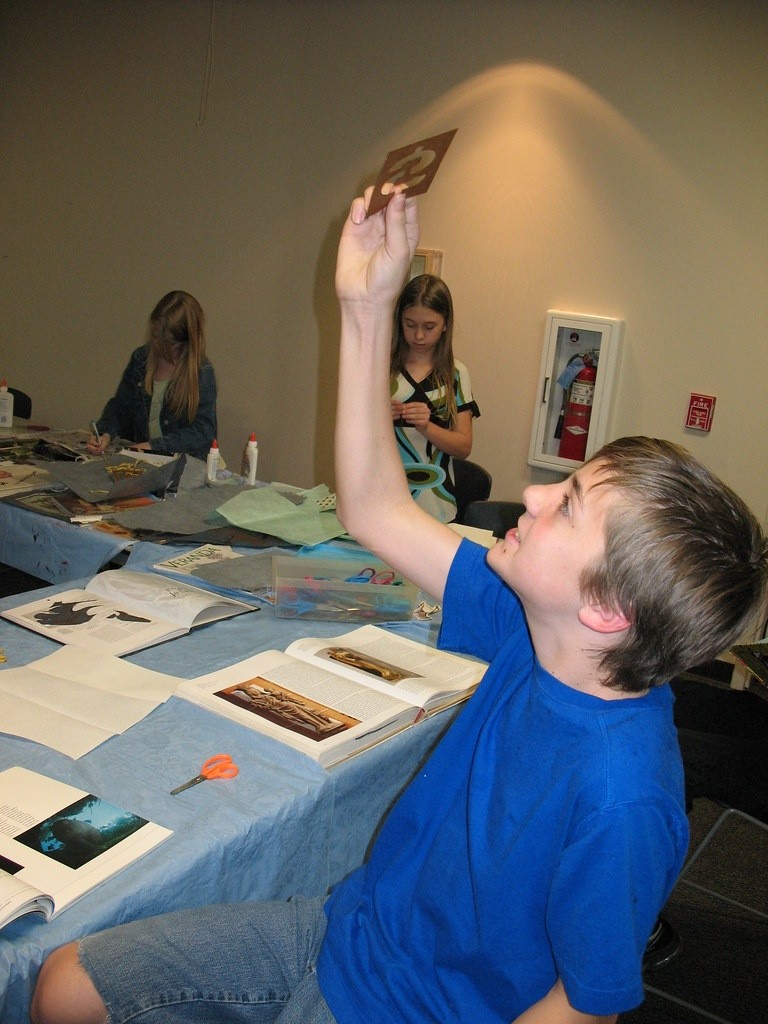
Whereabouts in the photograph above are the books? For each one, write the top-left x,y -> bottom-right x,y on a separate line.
0,766 -> 173,930
0,567 -> 260,658
173,624 -> 491,770
0,436 -> 272,600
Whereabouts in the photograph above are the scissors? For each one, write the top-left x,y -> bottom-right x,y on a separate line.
276,568 -> 414,623
170,753 -> 239,795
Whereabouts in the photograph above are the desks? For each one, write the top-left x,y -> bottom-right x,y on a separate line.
0,448 -> 488,1023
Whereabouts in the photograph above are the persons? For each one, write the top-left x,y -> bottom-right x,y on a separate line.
30,184 -> 768,1024
86,291 -> 218,462
390,273 -> 480,523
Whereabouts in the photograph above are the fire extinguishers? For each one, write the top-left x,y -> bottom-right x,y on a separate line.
558,348 -> 599,462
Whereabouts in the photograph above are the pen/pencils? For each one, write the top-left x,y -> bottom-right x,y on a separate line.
92,420 -> 104,456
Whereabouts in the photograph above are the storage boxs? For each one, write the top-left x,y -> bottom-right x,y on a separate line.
272,553 -> 417,624
